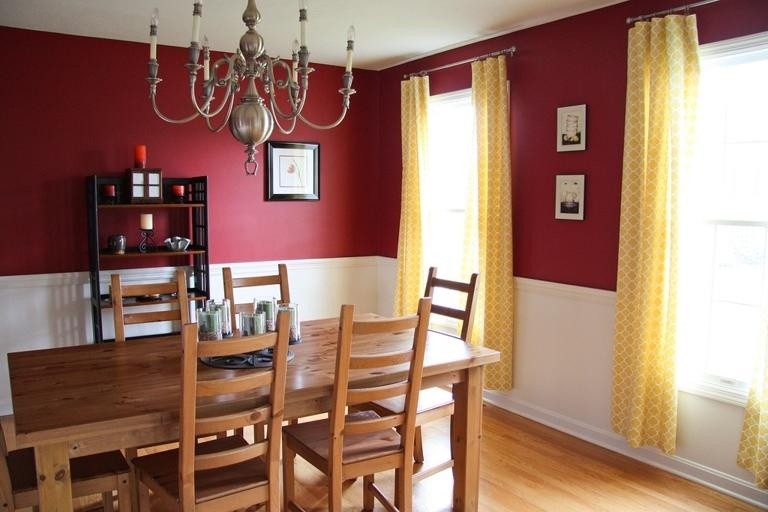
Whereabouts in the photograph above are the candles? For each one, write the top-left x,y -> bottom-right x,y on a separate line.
140,213 -> 153,229
102,185 -> 115,195
135,143 -> 146,167
172,186 -> 184,197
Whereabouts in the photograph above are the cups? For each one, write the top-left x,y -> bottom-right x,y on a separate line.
204,298 -> 233,337
252,296 -> 275,329
197,307 -> 222,342
277,302 -> 301,341
239,310 -> 267,353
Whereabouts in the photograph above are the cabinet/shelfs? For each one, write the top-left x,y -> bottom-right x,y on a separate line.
89,174 -> 211,344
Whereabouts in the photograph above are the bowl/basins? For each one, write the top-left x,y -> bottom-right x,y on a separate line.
165,235 -> 191,251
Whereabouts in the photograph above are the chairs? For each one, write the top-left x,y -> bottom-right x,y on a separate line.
348,266 -> 480,463
131,309 -> 290,512
111,272 -> 226,459
282,297 -> 432,512
220,264 -> 299,443
1,414 -> 130,511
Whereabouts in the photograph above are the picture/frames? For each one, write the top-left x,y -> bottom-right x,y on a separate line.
555,175 -> 585,221
264,140 -> 320,201
557,104 -> 586,152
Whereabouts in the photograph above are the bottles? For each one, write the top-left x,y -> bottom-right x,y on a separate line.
113,235 -> 127,255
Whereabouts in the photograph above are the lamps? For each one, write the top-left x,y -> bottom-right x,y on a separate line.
145,0 -> 357,175
